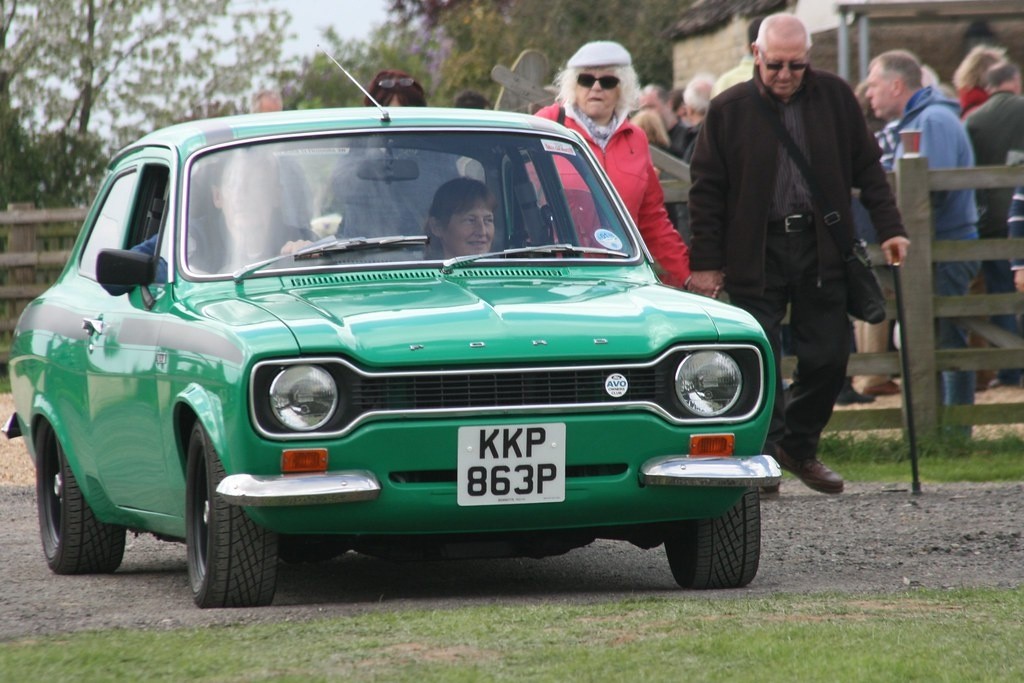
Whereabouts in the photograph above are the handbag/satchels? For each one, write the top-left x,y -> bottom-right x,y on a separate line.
845,237 -> 886,325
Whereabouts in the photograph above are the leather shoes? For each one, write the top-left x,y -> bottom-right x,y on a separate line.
774,446 -> 844,493
761,483 -> 780,493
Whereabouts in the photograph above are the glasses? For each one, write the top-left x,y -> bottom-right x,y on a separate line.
378,78 -> 415,88
758,48 -> 812,70
577,73 -> 621,90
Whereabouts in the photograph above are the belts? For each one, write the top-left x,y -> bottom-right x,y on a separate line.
767,212 -> 814,235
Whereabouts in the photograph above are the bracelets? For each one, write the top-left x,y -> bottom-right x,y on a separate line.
682,274 -> 693,288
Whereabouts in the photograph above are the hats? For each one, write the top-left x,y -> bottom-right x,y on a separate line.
566,40 -> 632,68
963,20 -> 993,38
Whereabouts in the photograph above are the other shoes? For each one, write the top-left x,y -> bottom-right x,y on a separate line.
863,380 -> 901,395
975,378 -> 1000,390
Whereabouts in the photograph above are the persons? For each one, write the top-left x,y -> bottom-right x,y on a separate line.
425,177 -> 497,260
131,144 -> 332,283
690,12 -> 911,497
533,41 -> 724,299
836,19 -> 1024,436
629,17 -> 766,249
334,71 -> 490,237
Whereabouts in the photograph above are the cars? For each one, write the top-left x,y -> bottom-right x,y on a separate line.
1,109 -> 781,607
